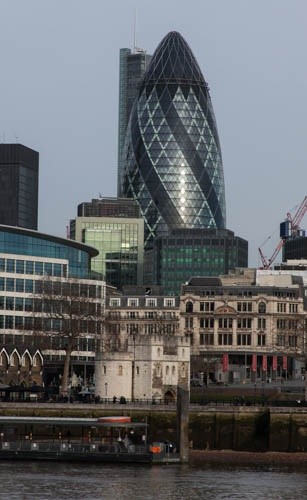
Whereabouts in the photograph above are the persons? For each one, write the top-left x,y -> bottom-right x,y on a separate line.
234,394 -> 252,406
61,395 -> 127,404
296,399 -> 306,406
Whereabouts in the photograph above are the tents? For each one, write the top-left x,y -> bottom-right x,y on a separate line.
0,383 -> 11,389
23,386 -> 48,400
0,385 -> 26,402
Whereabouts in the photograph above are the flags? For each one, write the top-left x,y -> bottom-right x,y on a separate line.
282,356 -> 288,370
222,354 -> 229,373
251,353 -> 257,373
262,354 -> 267,372
272,353 -> 277,371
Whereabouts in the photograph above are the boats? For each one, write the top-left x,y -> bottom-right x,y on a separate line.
1,415 -> 170,464
